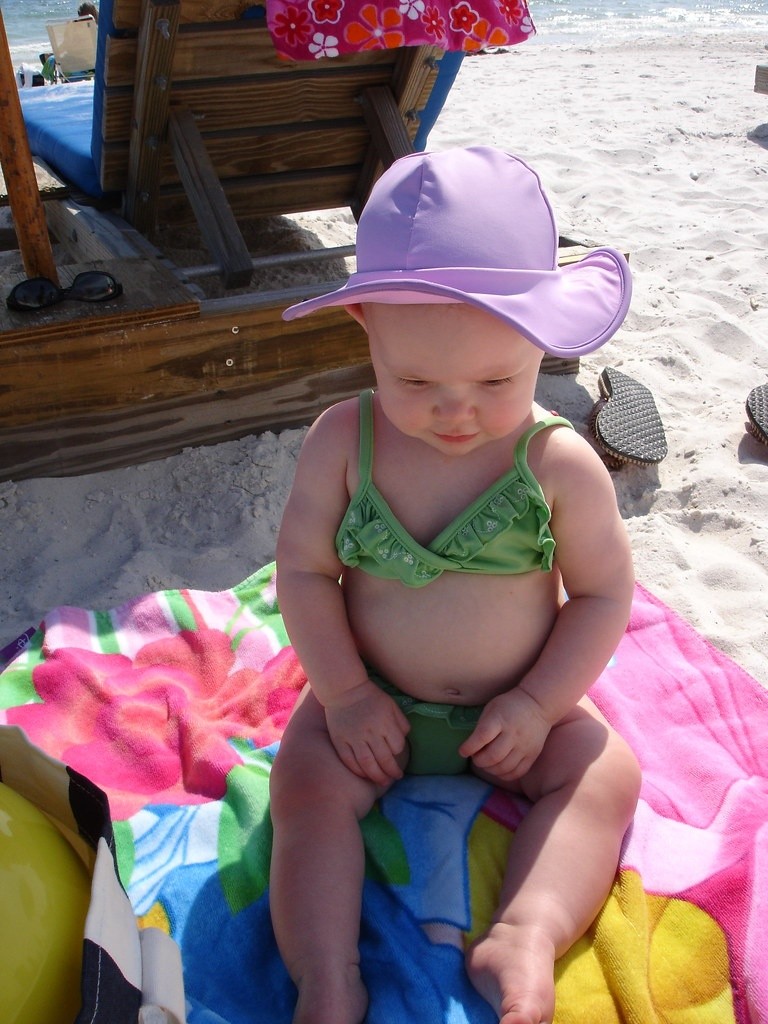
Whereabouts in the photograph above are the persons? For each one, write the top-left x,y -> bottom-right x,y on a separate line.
270,148 -> 642,1024
39,3 -> 99,75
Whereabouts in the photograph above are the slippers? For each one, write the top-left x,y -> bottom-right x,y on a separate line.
746,384 -> 768,445
589,366 -> 667,470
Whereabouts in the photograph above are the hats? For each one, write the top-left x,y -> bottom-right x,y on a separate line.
283,143 -> 632,357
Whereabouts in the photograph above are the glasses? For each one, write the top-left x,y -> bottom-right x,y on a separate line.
5,271 -> 123,311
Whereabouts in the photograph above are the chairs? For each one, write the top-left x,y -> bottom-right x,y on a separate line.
45,13 -> 98,83
16,0 -> 467,313
